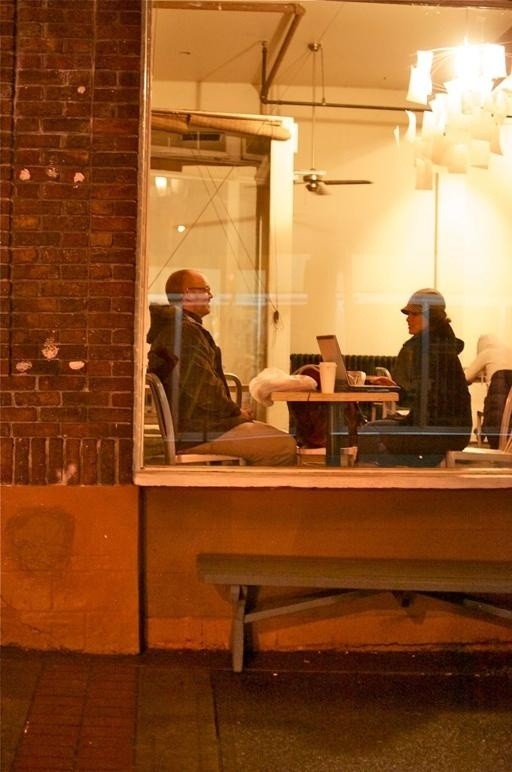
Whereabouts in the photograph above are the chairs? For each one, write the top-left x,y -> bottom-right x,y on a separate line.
445,381 -> 512,467
223,374 -> 242,409
146,373 -> 247,466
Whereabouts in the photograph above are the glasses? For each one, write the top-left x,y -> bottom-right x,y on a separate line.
188,286 -> 210,292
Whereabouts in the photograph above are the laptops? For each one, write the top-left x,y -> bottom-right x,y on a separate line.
316,334 -> 402,391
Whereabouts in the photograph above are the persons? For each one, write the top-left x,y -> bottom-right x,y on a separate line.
463,332 -> 511,441
356,288 -> 472,467
147,268 -> 299,465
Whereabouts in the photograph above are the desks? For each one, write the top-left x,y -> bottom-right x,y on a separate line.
271,392 -> 399,465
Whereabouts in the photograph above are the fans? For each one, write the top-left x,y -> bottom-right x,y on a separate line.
288,42 -> 375,197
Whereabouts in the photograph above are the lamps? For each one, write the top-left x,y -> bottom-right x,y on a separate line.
391,27 -> 512,194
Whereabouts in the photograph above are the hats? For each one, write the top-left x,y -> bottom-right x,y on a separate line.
401,288 -> 446,313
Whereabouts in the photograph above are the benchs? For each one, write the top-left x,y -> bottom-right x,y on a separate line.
198,554 -> 512,672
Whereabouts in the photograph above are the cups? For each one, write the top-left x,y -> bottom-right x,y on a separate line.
319,361 -> 337,393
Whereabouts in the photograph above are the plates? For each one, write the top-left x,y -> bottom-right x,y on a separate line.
351,385 -> 401,392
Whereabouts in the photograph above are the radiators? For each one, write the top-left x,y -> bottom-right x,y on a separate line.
290,353 -> 397,378
